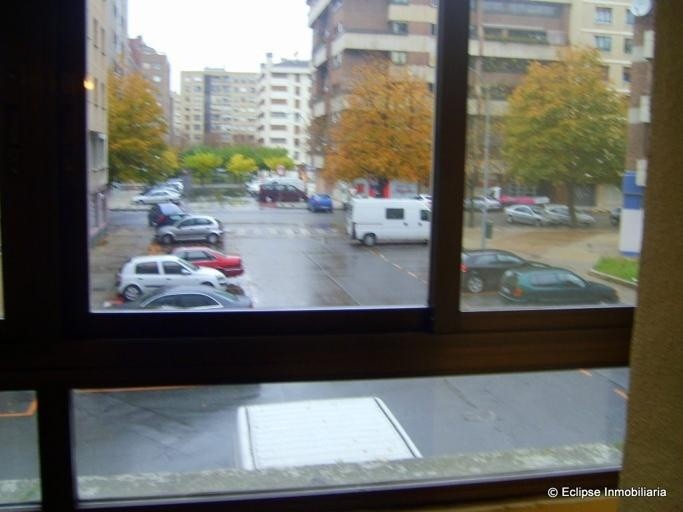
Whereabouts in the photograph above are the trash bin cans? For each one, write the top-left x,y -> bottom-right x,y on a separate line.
484,221 -> 495,239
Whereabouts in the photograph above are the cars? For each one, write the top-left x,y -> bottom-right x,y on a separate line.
258,183 -> 333,212
103,178 -> 255,310
463,193 -> 595,227
460,249 -> 620,307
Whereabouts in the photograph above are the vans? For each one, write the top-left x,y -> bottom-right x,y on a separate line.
345,194 -> 432,246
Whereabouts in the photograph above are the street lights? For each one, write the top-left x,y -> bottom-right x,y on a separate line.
467,64 -> 492,250
